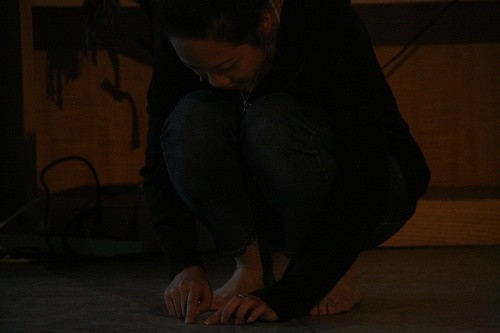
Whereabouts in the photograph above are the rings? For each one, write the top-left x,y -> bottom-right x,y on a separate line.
237,294 -> 247,299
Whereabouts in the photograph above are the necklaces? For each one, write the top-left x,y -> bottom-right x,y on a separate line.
237,42 -> 267,113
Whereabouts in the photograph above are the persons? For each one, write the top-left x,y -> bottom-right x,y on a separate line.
130,0 -> 431,326
